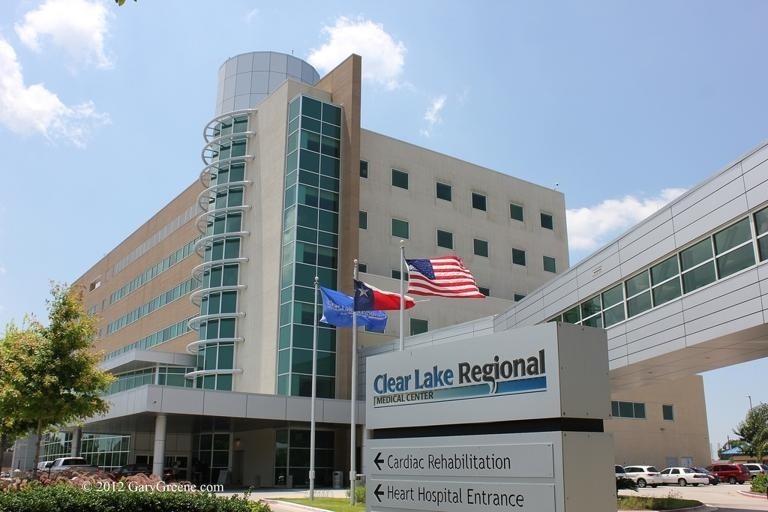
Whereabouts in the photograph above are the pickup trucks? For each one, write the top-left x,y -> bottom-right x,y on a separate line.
48,456 -> 100,478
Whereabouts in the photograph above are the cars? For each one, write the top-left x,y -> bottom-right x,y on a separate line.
615,465 -> 627,480
690,465 -> 719,485
623,465 -> 663,488
661,466 -> 709,486
36,460 -> 54,472
111,464 -> 173,483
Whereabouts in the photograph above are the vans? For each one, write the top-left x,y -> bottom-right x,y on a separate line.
705,462 -> 750,484
743,462 -> 768,480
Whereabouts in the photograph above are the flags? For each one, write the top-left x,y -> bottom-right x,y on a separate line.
319,287 -> 388,334
405,256 -> 485,299
353,278 -> 415,311
